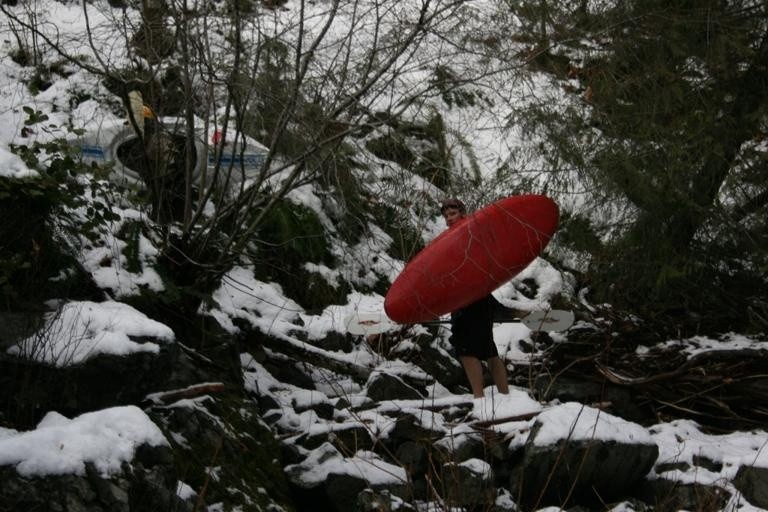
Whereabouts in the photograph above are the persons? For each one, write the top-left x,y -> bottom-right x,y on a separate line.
127,88 -> 176,226
441,196 -> 510,397
